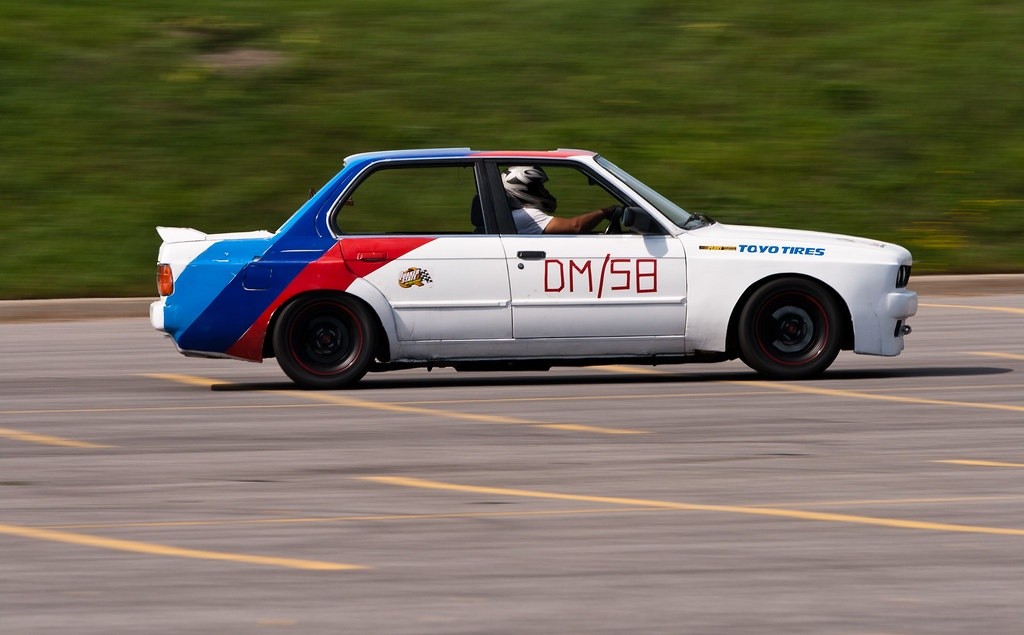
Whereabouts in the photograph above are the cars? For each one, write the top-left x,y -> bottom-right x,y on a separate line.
149,144 -> 918,391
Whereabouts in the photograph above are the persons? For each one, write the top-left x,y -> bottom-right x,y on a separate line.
500,165 -> 622,234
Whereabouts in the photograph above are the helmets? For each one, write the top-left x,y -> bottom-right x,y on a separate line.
500,166 -> 557,213
